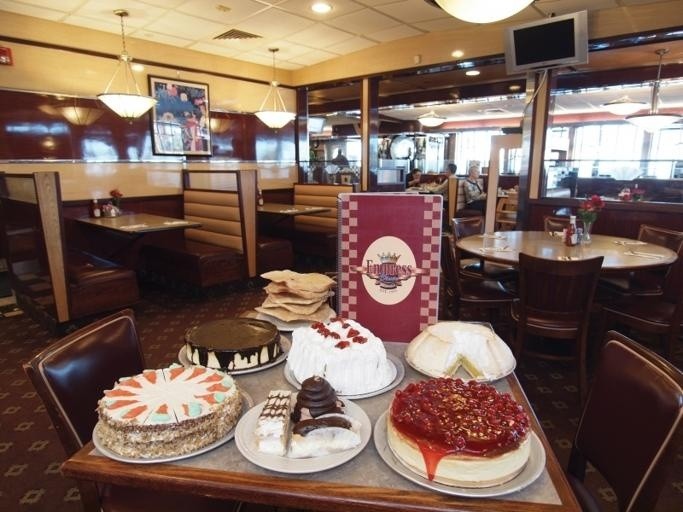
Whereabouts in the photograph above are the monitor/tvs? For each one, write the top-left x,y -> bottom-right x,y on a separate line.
505,9 -> 588,76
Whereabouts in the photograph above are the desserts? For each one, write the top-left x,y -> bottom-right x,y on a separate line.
286,412 -> 362,460
252,389 -> 293,456
291,375 -> 344,424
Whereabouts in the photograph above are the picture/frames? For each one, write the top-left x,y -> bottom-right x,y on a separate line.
147,74 -> 212,157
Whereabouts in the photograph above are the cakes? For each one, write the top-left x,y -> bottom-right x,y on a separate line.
287,315 -> 391,395
385,377 -> 533,489
185,317 -> 283,372
94,366 -> 245,462
407,321 -> 516,381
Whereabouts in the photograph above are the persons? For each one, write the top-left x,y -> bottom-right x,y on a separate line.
463,165 -> 485,215
405,167 -> 421,187
426,163 -> 456,211
331,150 -> 350,169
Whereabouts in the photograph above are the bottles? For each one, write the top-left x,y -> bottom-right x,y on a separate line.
90,199 -> 102,218
561,229 -> 568,243
577,228 -> 584,245
257,188 -> 264,206
566,214 -> 579,246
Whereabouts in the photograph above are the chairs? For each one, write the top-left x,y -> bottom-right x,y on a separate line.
21,306 -> 263,509
560,326 -> 682,510
406,173 -> 683,402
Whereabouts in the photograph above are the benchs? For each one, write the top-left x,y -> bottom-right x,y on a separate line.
143,167 -> 360,294
1,170 -> 139,333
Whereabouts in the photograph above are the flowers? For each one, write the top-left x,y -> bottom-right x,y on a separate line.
108,189 -> 123,204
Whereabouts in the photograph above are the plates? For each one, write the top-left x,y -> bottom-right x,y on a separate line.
233,396 -> 371,473
89,387 -> 253,466
173,335 -> 290,376
373,406 -> 549,501
284,349 -> 409,400
403,339 -> 518,385
255,309 -> 337,334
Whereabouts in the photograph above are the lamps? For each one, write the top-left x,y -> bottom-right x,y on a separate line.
53,97 -> 105,126
209,112 -> 234,135
417,112 -> 446,127
96,10 -> 158,125
603,96 -> 647,117
252,48 -> 296,134
623,48 -> 682,134
